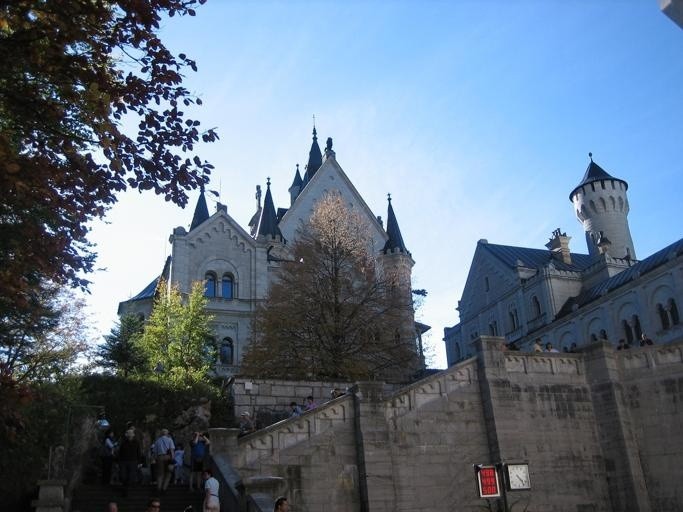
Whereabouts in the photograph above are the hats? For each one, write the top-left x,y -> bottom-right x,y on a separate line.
241,411 -> 250,417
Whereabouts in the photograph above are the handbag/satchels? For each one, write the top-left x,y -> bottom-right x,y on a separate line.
167,449 -> 172,455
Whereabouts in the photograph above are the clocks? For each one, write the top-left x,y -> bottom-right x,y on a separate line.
506,463 -> 532,491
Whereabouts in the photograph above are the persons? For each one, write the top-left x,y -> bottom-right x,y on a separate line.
534,337 -> 653,352
102,388 -> 347,512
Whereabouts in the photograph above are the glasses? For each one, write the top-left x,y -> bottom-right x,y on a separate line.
151,505 -> 161,508
